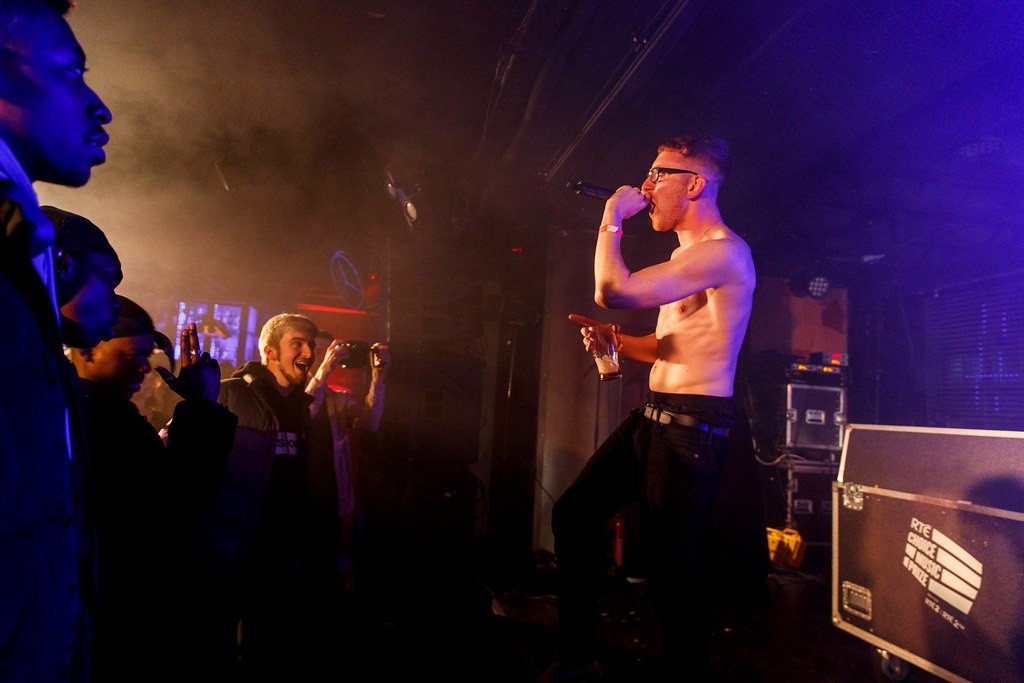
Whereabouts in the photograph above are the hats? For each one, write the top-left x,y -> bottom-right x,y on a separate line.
113,295 -> 153,336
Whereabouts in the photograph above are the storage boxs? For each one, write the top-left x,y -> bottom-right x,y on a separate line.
749,381 -> 845,556
829,423 -> 1024,683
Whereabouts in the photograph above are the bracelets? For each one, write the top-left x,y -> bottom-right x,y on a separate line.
597,224 -> 624,239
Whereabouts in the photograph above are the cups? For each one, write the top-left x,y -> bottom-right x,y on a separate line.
587,323 -> 622,381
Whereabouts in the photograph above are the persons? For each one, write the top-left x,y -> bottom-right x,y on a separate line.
553,134 -> 755,683
1,0 -> 391,682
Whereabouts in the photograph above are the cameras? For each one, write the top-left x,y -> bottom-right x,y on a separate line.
341,341 -> 370,369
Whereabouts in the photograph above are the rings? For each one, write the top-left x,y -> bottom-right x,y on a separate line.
190,349 -> 201,355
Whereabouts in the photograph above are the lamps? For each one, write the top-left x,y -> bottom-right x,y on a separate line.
803,262 -> 832,297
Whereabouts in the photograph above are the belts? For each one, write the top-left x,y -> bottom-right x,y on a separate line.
643,403 -> 730,437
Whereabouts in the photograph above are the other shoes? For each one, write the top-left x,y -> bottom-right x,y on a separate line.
534,658 -> 595,683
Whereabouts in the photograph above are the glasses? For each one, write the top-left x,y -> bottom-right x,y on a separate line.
647,167 -> 697,183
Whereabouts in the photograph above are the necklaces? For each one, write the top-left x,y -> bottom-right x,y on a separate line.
700,218 -> 726,242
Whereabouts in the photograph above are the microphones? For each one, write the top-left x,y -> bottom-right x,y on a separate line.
567,181 -> 652,214
